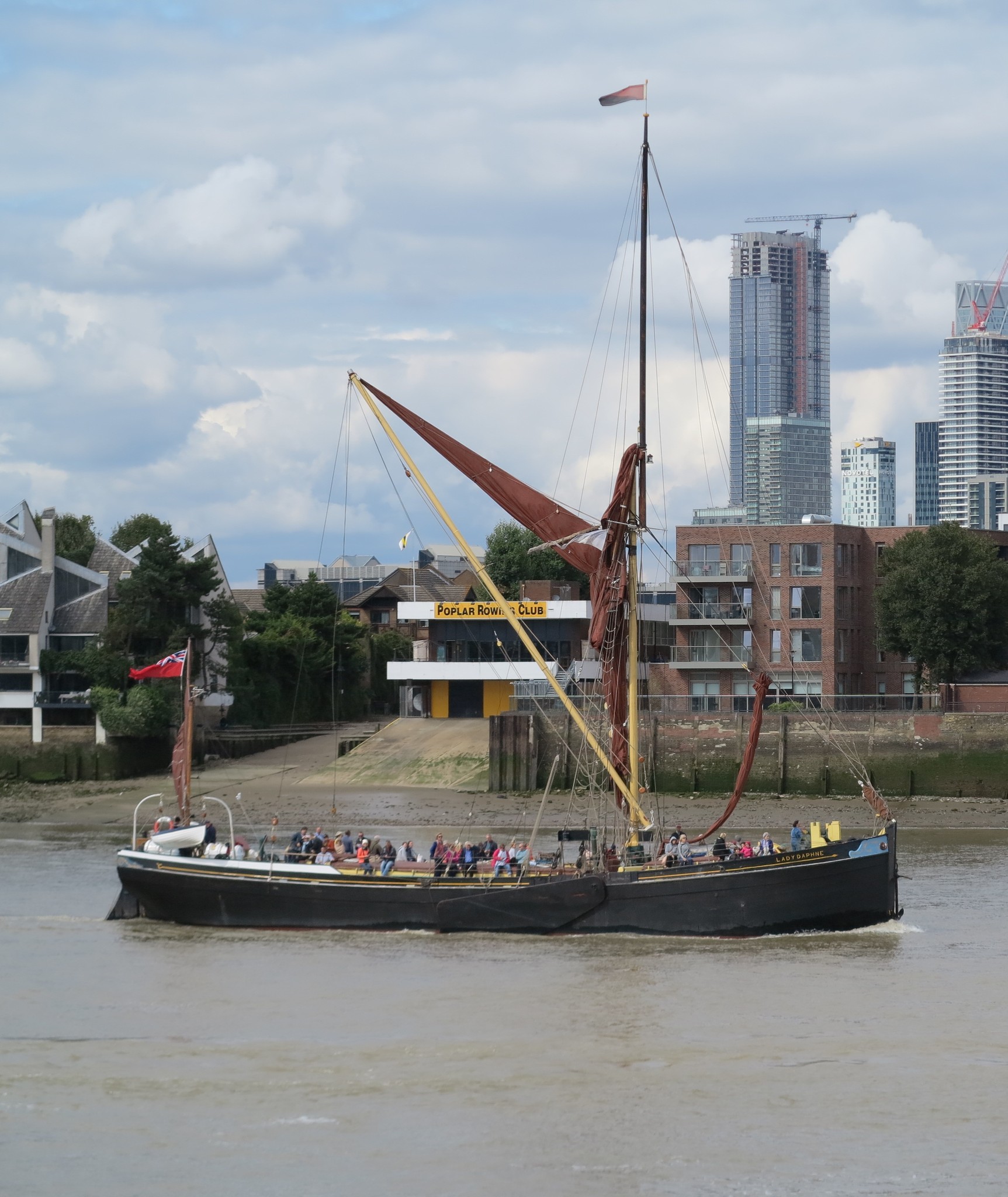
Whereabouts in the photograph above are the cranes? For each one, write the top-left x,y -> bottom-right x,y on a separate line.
966,252 -> 1008,332
742,213 -> 857,421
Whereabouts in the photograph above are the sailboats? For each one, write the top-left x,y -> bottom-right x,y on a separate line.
108,81 -> 903,935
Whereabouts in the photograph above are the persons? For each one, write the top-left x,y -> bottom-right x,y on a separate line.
713,831 -> 773,862
698,834 -> 706,846
173,814 -> 262,861
582,844 -> 617,861
284,827 -> 418,877
791,820 -> 831,851
430,832 -> 532,878
662,823 -> 693,869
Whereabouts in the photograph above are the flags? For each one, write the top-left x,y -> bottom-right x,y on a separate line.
599,79 -> 649,107
128,647 -> 188,679
